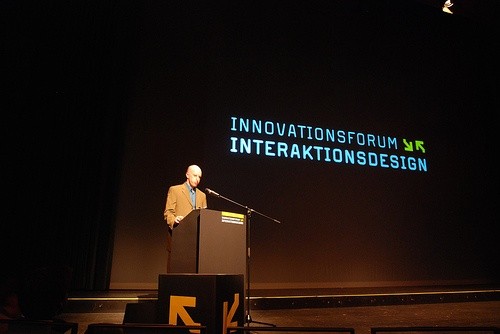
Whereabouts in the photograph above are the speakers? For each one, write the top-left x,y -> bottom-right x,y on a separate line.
122,303 -> 158,324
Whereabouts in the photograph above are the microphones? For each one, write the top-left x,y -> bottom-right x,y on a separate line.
205,188 -> 219,197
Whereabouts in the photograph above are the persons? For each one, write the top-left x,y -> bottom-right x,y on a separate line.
164,164 -> 208,235
